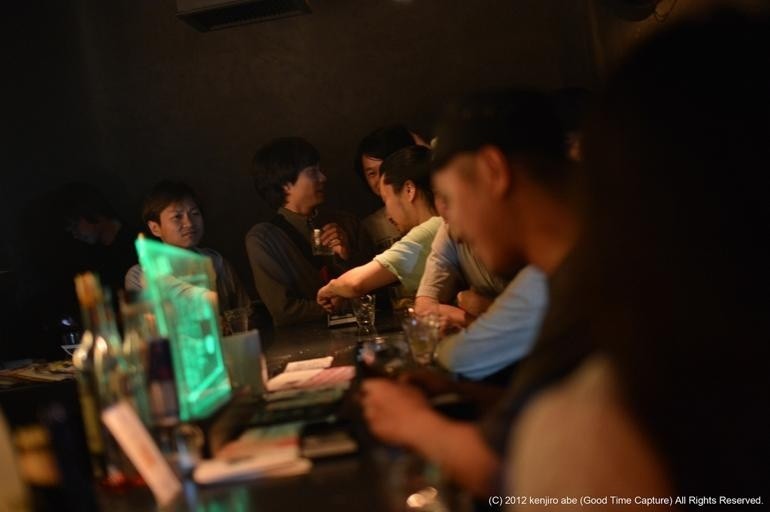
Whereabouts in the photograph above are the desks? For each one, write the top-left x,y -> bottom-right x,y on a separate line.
198,319 -> 471,511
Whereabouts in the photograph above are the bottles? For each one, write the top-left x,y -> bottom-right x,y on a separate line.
72,264 -> 156,512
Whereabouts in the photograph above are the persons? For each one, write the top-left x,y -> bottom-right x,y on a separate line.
55,87 -> 766,509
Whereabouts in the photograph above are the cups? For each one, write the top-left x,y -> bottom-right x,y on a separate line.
401,309 -> 442,368
224,307 -> 252,335
350,292 -> 377,335
311,229 -> 334,255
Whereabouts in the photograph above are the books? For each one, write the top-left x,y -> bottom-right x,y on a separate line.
0,358 -> 75,389
